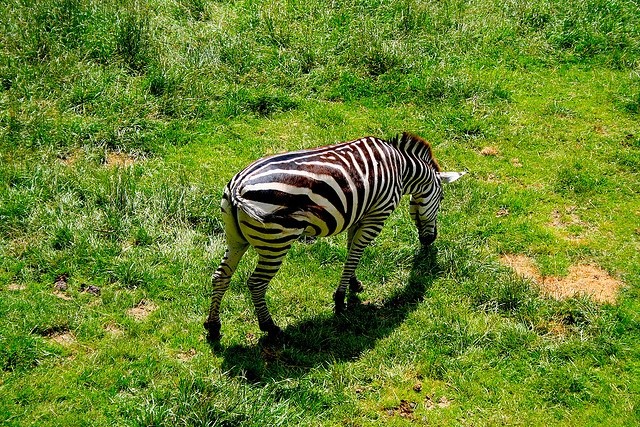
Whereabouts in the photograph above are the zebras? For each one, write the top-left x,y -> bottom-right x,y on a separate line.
201,129 -> 465,342
